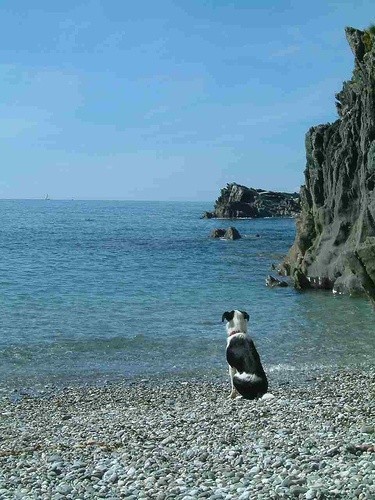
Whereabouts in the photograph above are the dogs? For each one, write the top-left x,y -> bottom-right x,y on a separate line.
220,309 -> 274,401
